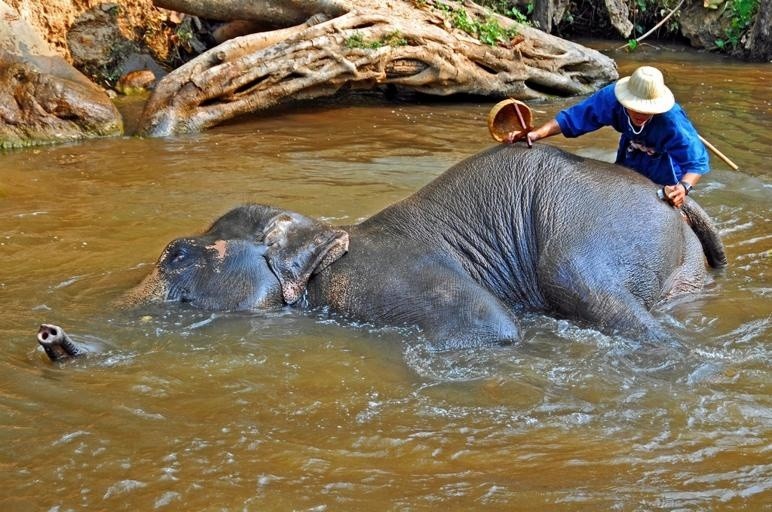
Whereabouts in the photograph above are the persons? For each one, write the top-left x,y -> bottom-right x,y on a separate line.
501,64 -> 711,209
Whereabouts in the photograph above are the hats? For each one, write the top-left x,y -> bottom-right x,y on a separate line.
614,65 -> 674,114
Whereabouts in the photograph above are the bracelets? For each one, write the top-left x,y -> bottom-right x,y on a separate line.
679,180 -> 693,195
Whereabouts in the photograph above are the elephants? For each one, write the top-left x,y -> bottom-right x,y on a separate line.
38,135 -> 727,371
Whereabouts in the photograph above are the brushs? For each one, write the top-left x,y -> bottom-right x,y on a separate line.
657,186 -> 675,201
